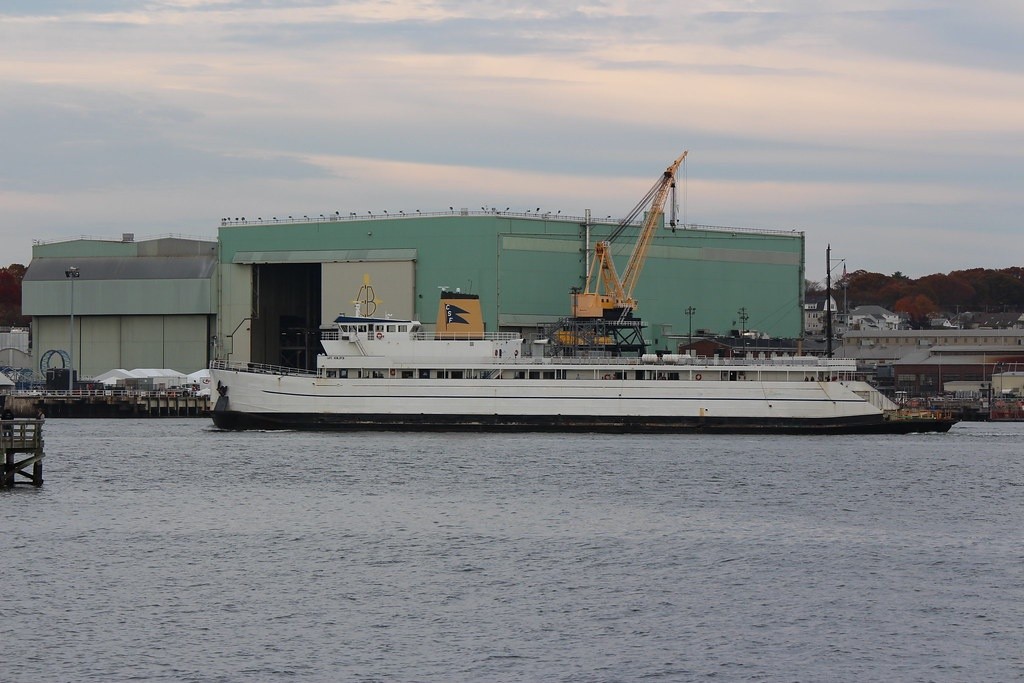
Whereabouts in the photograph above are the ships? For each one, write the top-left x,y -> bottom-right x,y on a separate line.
207,299 -> 903,428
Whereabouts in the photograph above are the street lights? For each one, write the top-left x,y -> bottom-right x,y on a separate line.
825,244 -> 833,357
842,263 -> 851,329
737,307 -> 750,357
65,265 -> 80,396
684,304 -> 696,356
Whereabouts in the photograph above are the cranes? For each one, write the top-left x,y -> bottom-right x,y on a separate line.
554,148 -> 693,319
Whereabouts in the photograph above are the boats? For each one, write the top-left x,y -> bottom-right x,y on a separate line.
886,409 -> 962,433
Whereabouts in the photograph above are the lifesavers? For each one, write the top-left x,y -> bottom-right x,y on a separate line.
376,333 -> 383,339
696,374 -> 702,380
604,374 -> 610,380
390,369 -> 395,375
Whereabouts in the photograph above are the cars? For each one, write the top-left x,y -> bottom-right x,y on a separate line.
166,385 -> 190,397
